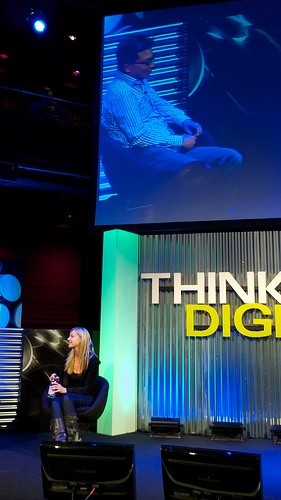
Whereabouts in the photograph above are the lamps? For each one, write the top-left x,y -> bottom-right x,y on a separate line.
148,417 -> 181,438
270,425 -> 281,444
210,421 -> 245,441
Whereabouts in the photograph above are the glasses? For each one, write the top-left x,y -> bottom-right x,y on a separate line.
126,53 -> 156,66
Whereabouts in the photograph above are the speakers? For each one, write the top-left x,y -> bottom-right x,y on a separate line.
211,422 -> 242,437
150,417 -> 180,434
273,424 -> 281,439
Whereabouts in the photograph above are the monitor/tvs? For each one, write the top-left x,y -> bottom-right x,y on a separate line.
40,441 -> 137,500
161,444 -> 263,500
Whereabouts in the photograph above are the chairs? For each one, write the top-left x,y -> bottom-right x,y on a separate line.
43,376 -> 109,440
100,116 -> 211,210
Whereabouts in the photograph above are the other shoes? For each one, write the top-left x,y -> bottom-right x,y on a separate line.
50,418 -> 66,441
65,415 -> 78,436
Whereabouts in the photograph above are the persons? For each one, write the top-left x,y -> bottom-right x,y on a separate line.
48,327 -> 102,441
102,33 -> 244,183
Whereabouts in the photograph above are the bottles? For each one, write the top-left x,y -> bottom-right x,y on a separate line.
47,376 -> 56,399
